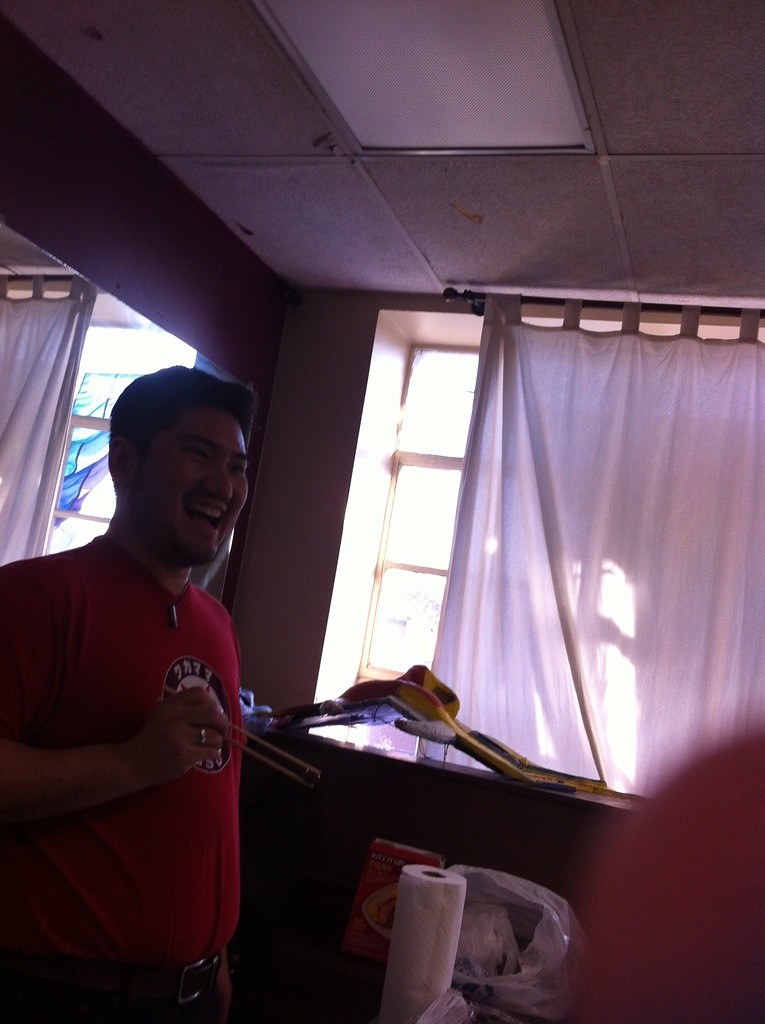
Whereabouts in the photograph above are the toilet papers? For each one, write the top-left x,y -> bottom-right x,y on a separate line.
376,865 -> 467,1024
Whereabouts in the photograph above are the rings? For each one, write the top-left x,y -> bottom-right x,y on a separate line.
199,727 -> 206,745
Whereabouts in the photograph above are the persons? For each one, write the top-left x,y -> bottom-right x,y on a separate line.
562,726 -> 765,1024
0,368 -> 252,1023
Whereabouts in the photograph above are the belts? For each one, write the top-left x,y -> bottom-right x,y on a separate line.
18,951 -> 224,1007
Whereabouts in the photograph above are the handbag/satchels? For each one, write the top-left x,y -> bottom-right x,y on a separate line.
441,863 -> 588,1019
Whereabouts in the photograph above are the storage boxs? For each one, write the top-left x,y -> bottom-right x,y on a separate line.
339,838 -> 447,966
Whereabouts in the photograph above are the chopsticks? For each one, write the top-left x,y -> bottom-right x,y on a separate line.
155,685 -> 320,790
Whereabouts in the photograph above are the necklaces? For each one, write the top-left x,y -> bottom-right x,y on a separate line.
168,579 -> 189,629
